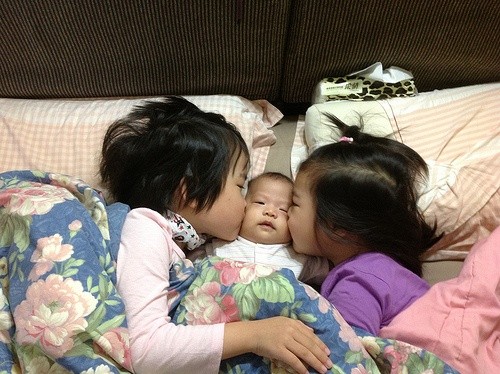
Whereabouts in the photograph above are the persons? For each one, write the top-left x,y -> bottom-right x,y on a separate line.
186,172 -> 335,288
288,112 -> 447,338
98,95 -> 333,374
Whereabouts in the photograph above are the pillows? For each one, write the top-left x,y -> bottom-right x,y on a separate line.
0,94 -> 284,206
291,83 -> 500,261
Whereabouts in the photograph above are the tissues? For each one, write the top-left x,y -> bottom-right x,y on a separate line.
312,61 -> 418,104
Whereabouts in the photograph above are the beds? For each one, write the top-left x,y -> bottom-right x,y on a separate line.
0,0 -> 500,374
